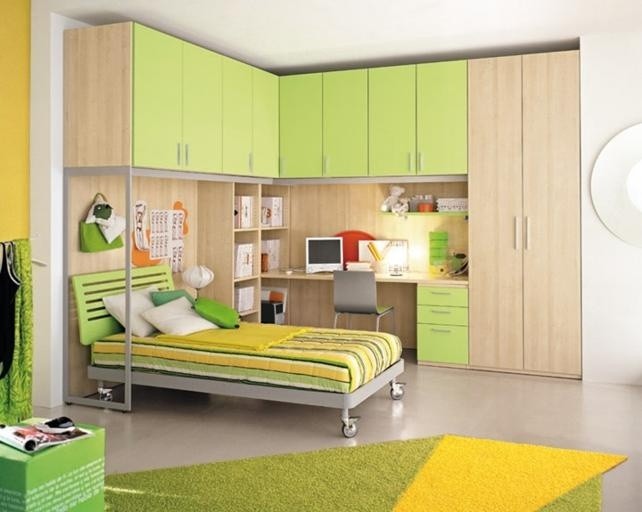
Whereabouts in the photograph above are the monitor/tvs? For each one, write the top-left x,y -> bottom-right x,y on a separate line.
306,237 -> 344,273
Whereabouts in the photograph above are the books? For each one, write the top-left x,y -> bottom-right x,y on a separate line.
236,196 -> 284,313
345,260 -> 375,274
1,423 -> 92,453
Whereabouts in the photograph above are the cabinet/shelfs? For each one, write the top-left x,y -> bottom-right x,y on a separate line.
279,67 -> 367,178
0,417 -> 106,512
368,60 -> 467,176
224,57 -> 280,181
65,20 -> 228,177
260,183 -> 290,271
196,183 -> 259,323
468,48 -> 582,380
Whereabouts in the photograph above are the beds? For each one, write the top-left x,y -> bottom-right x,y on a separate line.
70,265 -> 407,438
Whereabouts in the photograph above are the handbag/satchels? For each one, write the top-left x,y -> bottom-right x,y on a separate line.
79,193 -> 123,252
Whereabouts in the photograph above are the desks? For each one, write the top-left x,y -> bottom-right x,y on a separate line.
262,266 -> 469,366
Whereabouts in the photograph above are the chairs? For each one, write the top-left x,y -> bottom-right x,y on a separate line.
332,271 -> 396,339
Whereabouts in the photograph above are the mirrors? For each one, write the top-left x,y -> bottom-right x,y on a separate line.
589,122 -> 642,254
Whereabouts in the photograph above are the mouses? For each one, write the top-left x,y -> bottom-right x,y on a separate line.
286,270 -> 292,274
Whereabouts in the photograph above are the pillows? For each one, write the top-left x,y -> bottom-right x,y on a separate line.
140,297 -> 219,336
194,296 -> 240,329
103,284 -> 162,337
149,289 -> 194,311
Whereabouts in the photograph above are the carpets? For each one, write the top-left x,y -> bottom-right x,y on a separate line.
104,435 -> 631,511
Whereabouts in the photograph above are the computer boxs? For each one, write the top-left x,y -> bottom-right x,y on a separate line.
261,300 -> 284,324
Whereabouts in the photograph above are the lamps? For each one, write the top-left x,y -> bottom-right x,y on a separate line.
180,264 -> 215,300
